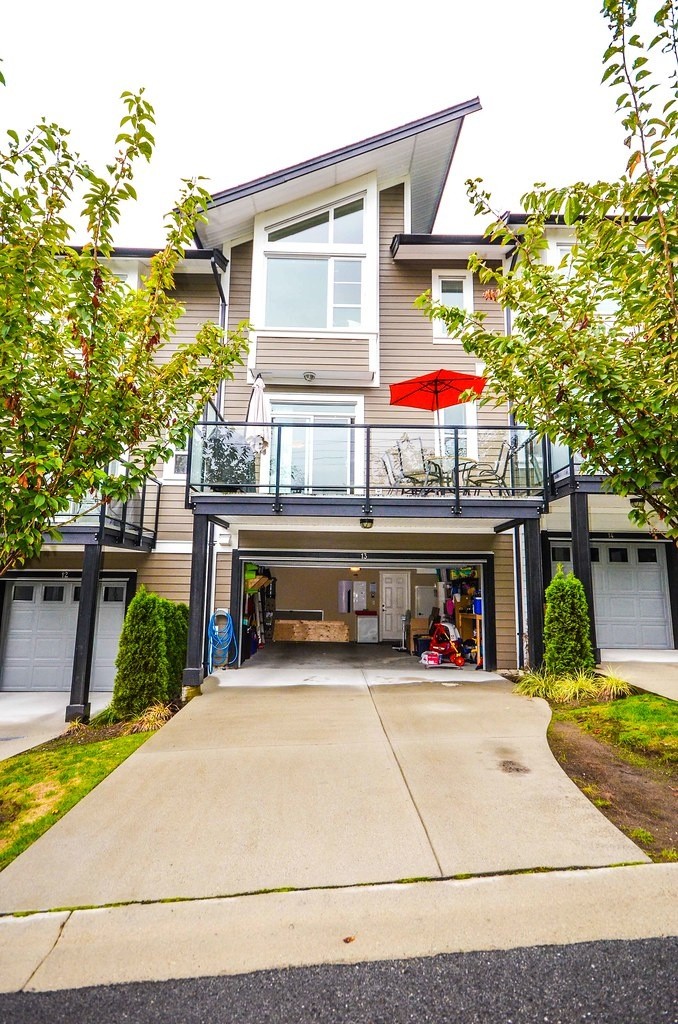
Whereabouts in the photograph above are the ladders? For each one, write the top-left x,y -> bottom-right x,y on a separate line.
254,588 -> 265,644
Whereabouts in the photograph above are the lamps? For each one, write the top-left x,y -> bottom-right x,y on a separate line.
630,498 -> 646,509
349,568 -> 361,571
360,519 -> 374,528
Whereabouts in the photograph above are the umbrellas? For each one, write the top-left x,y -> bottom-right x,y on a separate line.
243,373 -> 273,492
389,368 -> 490,467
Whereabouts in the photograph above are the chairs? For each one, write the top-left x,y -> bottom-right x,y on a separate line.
380,452 -> 418,496
474,468 -> 506,495
462,439 -> 516,496
397,436 -> 446,497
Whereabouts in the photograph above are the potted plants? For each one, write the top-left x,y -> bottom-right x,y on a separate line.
199,421 -> 268,493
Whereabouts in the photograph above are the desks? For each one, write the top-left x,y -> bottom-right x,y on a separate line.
427,457 -> 477,497
459,612 -> 483,665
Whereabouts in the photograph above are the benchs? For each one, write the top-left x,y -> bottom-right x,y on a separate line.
405,617 -> 430,655
